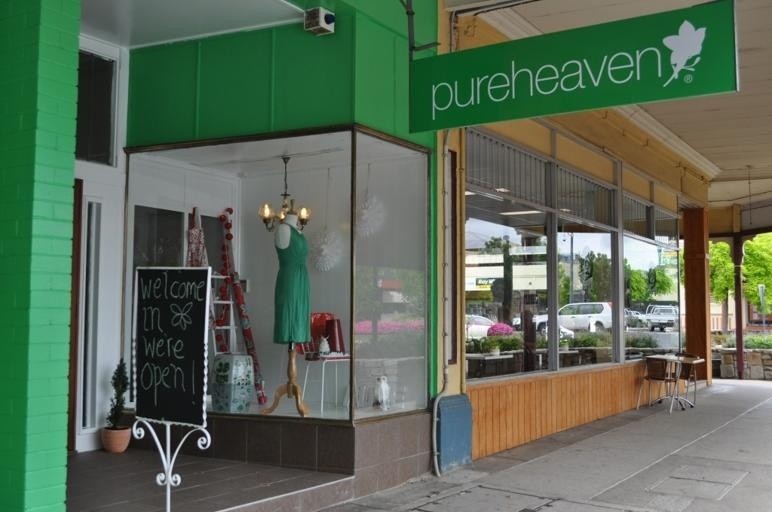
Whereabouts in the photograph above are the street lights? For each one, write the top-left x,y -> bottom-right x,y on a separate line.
562,233 -> 573,303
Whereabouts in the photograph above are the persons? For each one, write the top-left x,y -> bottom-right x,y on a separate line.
274,210 -> 312,344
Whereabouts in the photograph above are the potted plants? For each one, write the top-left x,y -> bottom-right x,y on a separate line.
103,357 -> 133,454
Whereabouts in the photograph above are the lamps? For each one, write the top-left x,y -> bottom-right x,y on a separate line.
257,156 -> 312,232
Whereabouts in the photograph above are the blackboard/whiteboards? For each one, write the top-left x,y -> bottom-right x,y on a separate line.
132,266 -> 212,429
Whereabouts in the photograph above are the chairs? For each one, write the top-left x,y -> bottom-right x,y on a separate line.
636,353 -> 706,415
302,313 -> 350,414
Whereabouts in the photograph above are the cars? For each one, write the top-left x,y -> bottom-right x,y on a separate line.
464,300 -> 679,342
